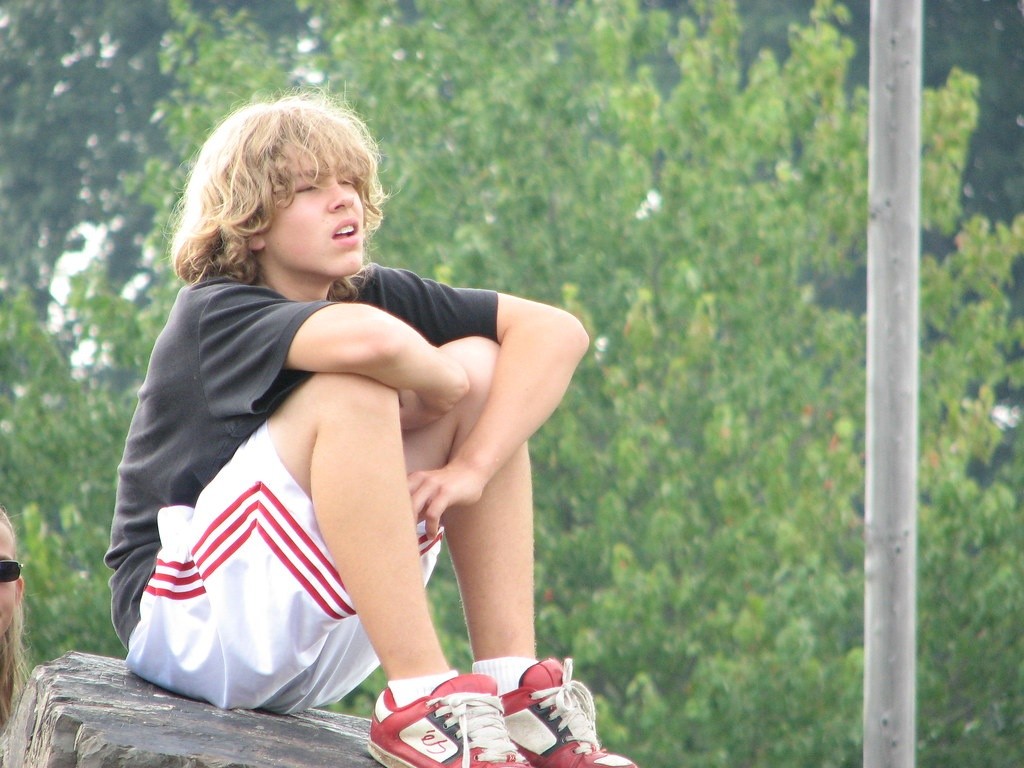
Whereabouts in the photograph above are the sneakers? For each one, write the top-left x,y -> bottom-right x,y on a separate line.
367,676 -> 535,767
497,657 -> 637,767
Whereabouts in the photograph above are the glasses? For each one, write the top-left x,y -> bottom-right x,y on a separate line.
0,559 -> 23,582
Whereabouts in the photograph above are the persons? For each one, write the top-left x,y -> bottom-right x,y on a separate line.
106,98 -> 637,768
0,507 -> 24,768
